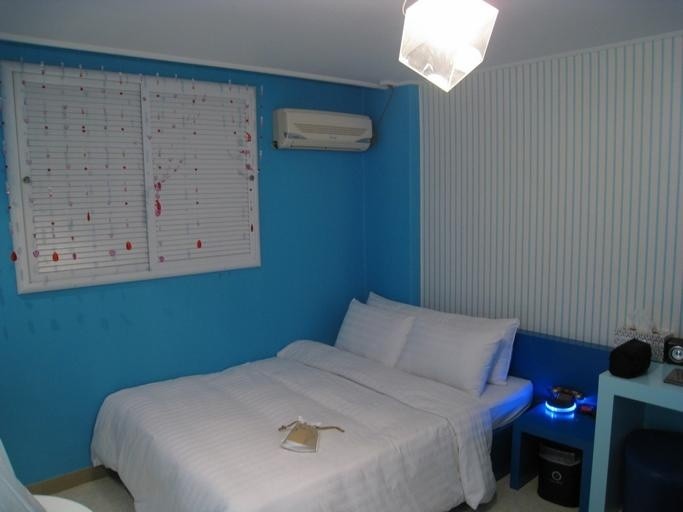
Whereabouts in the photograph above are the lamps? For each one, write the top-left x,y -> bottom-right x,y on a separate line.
397,0 -> 499,92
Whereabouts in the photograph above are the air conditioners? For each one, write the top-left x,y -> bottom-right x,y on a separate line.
272,107 -> 372,152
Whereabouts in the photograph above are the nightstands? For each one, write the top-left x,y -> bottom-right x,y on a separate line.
510,402 -> 595,512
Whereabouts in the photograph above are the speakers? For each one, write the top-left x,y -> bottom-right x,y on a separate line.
664,337 -> 683,365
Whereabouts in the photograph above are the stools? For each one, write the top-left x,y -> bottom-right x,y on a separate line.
623,429 -> 682,512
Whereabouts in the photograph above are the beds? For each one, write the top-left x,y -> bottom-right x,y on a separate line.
89,291 -> 534,512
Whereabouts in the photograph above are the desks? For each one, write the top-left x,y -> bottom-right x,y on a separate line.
591,362 -> 683,512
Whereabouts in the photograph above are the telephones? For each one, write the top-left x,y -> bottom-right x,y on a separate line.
545,386 -> 584,413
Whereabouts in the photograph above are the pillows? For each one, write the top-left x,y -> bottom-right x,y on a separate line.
334,296 -> 416,369
394,319 -> 501,399
366,292 -> 520,385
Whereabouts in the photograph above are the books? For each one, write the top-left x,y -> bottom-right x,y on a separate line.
664,368 -> 683,385
